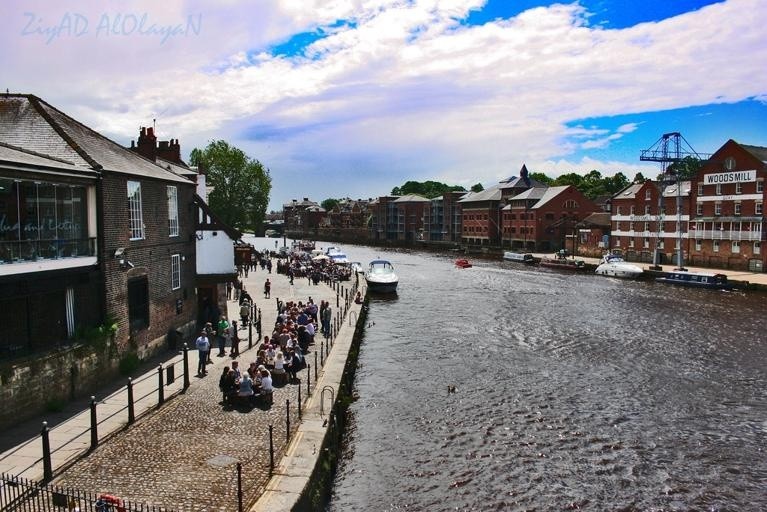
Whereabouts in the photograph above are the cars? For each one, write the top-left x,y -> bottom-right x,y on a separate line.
556,248 -> 572,256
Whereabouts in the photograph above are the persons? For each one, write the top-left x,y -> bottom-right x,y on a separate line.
190,238 -> 366,412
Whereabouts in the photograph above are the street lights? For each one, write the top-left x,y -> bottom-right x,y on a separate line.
282,232 -> 288,247
571,218 -> 577,259
524,209 -> 527,249
509,208 -> 512,250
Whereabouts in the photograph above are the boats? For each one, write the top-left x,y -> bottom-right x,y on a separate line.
540,255 -> 588,271
454,258 -> 472,269
292,239 -> 364,276
595,254 -> 644,279
501,250 -> 542,264
656,269 -> 736,291
365,256 -> 398,294
266,230 -> 281,239
